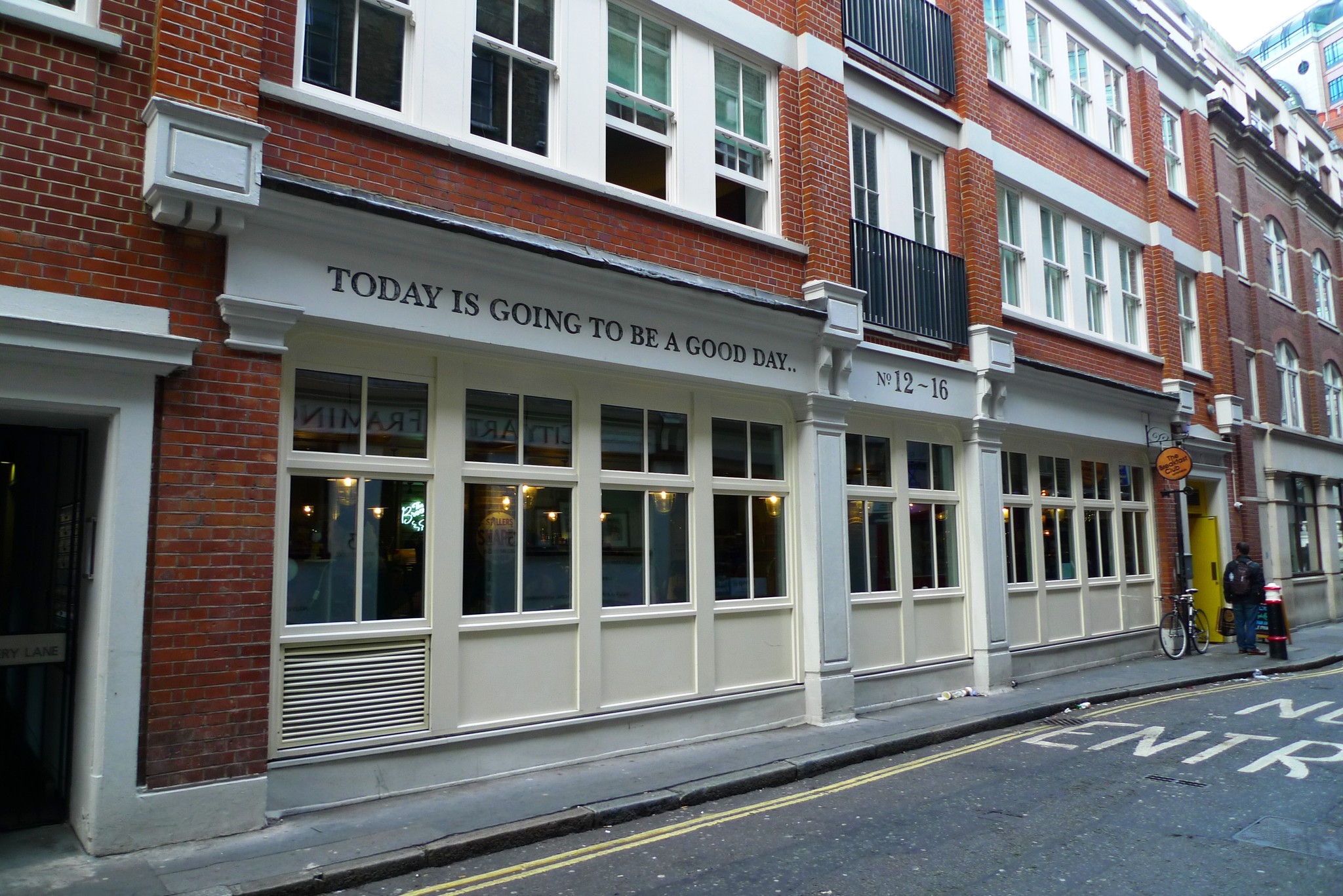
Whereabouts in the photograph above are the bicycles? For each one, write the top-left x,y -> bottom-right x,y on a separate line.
1153,588 -> 1210,660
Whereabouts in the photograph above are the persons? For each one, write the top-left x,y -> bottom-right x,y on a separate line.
1223,542 -> 1267,656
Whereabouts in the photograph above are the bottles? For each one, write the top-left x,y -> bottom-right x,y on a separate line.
541,528 -> 546,544
1228,572 -> 1234,581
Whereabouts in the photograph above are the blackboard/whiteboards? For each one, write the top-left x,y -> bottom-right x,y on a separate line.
1255,595 -> 1292,644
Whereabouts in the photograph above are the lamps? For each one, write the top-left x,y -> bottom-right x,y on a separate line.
600,511 -> 611,521
649,492 -> 677,513
325,477 -> 372,506
758,496 -> 784,518
543,493 -> 565,522
501,488 -> 512,510
367,507 -> 389,519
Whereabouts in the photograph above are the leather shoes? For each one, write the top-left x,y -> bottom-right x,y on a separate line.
1248,647 -> 1266,655
1239,649 -> 1247,653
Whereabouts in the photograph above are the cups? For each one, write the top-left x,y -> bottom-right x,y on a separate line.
960,687 -> 973,697
941,692 -> 952,700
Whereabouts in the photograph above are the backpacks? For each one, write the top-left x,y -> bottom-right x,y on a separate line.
1229,559 -> 1253,598
